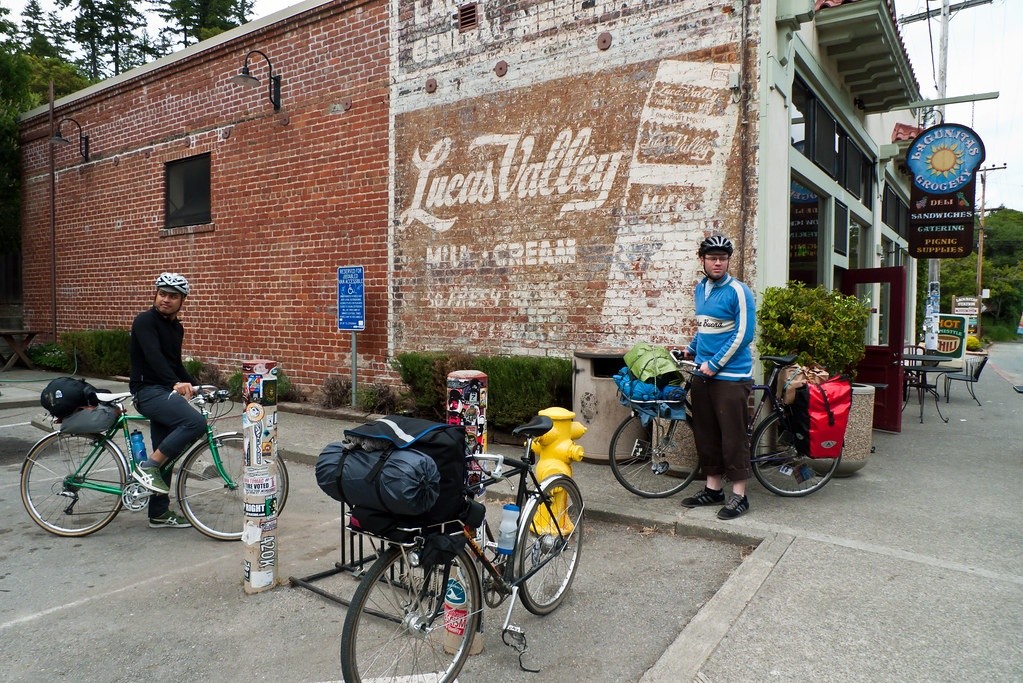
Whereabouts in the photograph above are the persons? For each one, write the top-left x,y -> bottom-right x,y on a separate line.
676,235 -> 756,520
128,273 -> 207,529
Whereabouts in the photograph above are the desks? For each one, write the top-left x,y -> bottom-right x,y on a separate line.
902,355 -> 953,424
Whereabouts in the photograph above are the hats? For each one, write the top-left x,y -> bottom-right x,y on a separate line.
160,286 -> 181,294
707,250 -> 727,255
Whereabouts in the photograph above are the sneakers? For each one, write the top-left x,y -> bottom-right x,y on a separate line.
132,460 -> 170,494
718,493 -> 749,520
150,510 -> 192,528
680,488 -> 725,507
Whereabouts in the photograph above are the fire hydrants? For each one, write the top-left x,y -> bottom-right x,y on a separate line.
522,405 -> 589,538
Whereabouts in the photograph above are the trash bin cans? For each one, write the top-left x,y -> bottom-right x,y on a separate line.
572,349 -> 648,466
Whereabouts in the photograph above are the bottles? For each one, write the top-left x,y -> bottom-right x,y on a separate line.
129,429 -> 147,466
497,503 -> 520,554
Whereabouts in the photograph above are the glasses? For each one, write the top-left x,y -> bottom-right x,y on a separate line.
704,255 -> 730,262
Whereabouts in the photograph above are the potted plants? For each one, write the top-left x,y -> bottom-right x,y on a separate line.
761,281 -> 875,478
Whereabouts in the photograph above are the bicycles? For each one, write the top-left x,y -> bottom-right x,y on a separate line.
608,348 -> 845,500
18,384 -> 291,538
337,415 -> 587,683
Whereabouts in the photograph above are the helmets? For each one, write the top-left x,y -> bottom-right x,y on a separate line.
698,236 -> 733,258
155,273 -> 189,295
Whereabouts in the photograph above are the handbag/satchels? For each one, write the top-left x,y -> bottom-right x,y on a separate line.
791,374 -> 852,459
613,343 -> 686,412
774,363 -> 829,405
59,404 -> 120,434
315,415 -> 486,565
40,376 -> 113,418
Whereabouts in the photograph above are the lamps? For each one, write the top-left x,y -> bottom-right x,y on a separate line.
233,49 -> 282,111
49,118 -> 90,162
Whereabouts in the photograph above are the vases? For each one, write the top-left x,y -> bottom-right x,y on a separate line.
653,381 -> 703,477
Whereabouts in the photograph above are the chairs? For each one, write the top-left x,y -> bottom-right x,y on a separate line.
902,345 -> 987,407
